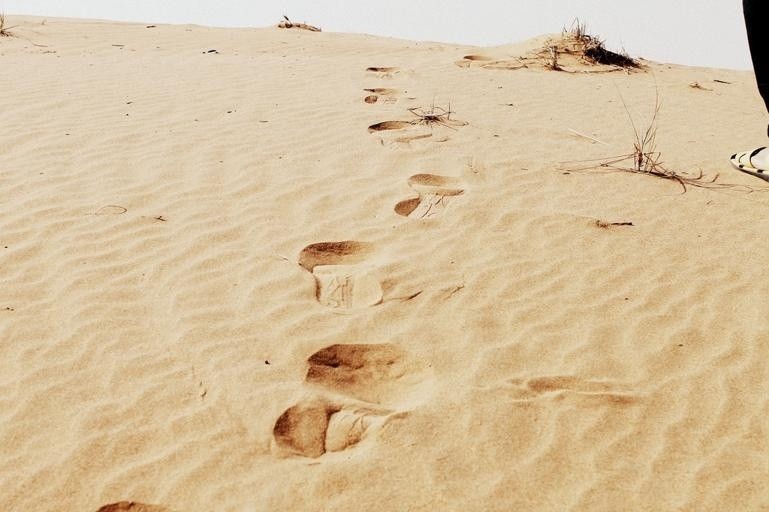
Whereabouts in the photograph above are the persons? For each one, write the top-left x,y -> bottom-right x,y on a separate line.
728,0 -> 767,181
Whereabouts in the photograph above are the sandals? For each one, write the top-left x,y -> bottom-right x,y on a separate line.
730,146 -> 769,181
